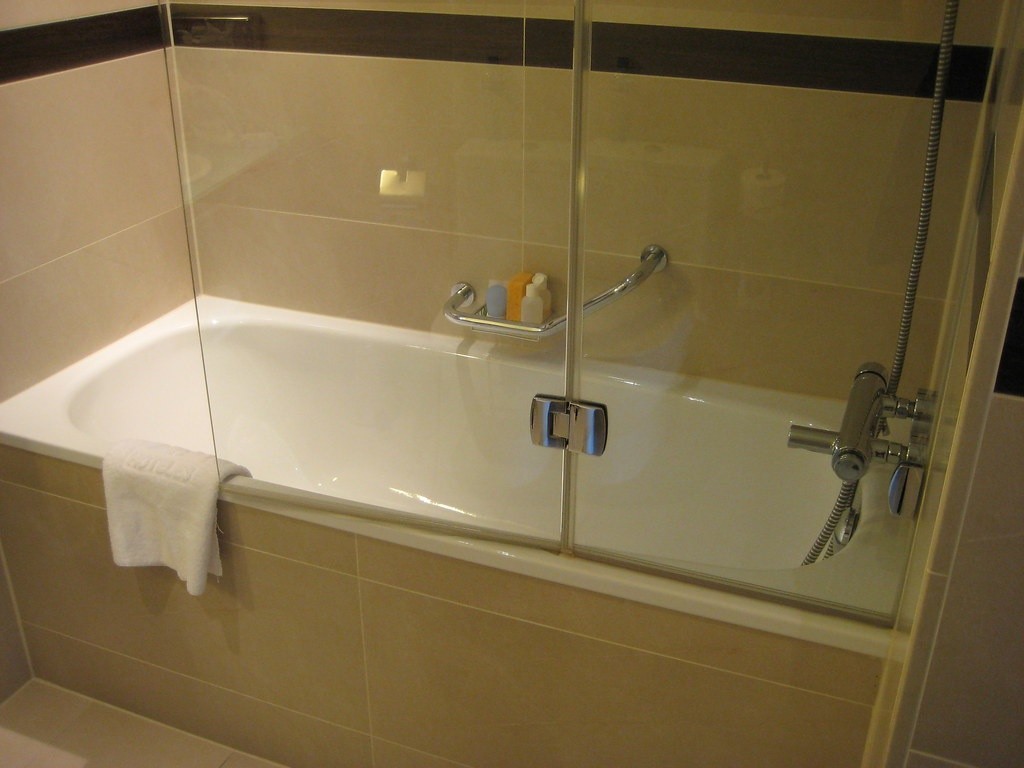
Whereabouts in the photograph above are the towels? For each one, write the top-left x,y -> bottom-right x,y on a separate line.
102,438 -> 253,597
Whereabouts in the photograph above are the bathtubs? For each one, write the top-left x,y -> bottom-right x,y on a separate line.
0,294 -> 938,768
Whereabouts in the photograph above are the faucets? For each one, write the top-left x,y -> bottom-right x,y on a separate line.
787,361 -> 939,481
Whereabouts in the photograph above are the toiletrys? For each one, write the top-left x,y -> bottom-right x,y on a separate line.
484,272 -> 552,327
